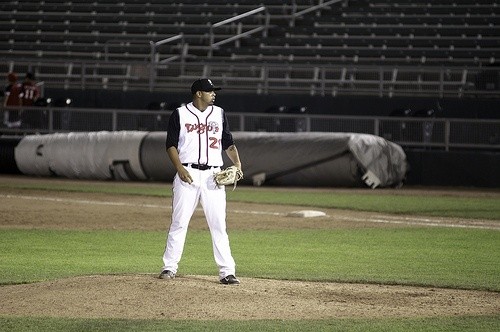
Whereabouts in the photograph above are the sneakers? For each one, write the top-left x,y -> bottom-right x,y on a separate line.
159,270 -> 175,279
219,275 -> 240,285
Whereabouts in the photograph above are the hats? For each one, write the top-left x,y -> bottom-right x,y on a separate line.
191,78 -> 221,92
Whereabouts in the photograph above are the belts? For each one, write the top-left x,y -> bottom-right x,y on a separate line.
182,163 -> 219,170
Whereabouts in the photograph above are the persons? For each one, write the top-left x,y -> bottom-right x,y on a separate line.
2,71 -> 41,129
158,78 -> 244,285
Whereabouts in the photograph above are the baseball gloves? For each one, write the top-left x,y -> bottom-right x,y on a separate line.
213,166 -> 243,191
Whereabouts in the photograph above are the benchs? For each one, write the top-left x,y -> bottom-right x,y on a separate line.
1,1 -> 500,97
